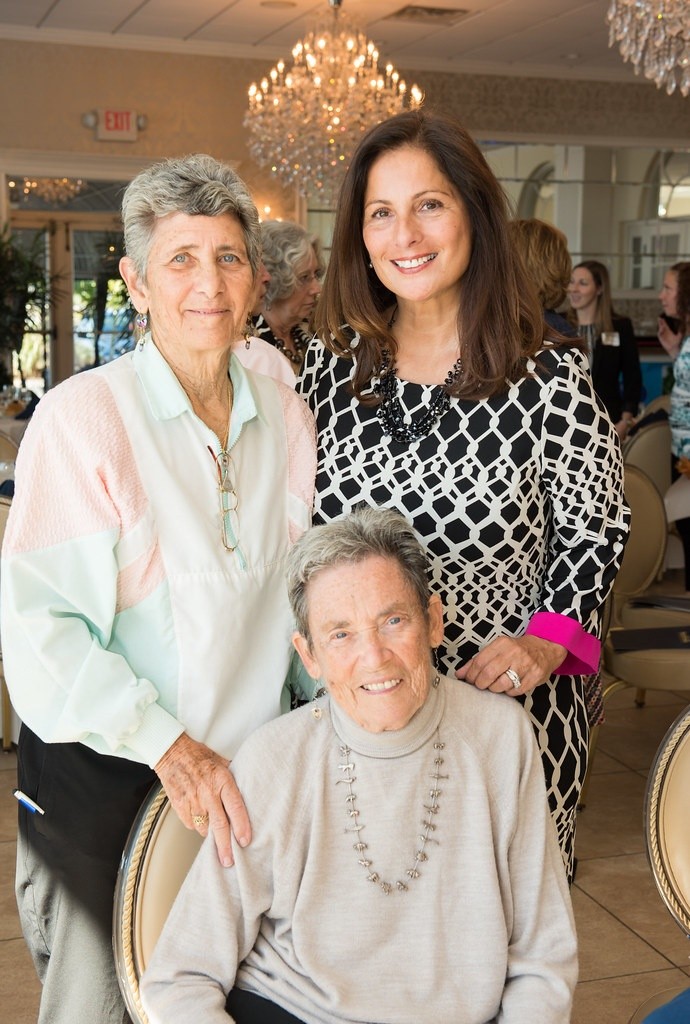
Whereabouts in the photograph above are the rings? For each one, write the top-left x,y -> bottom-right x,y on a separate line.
506,669 -> 521,689
191,815 -> 208,825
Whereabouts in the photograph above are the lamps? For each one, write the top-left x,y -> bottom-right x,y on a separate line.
242,0 -> 426,207
604,0 -> 690,98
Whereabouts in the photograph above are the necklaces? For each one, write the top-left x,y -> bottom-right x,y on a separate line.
273,333 -> 304,364
336,727 -> 449,893
374,305 -> 465,442
222,383 -> 231,454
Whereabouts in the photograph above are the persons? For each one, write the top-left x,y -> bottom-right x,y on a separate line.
246,220 -> 690,592
295,110 -> 630,883
1,155 -> 316,1024
137,506 -> 579,1024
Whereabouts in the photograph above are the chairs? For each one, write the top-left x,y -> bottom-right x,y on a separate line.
575,418 -> 690,1024
110,781 -> 205,1024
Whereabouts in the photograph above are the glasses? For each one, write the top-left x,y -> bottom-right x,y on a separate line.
208,445 -> 240,552
300,271 -> 322,286
